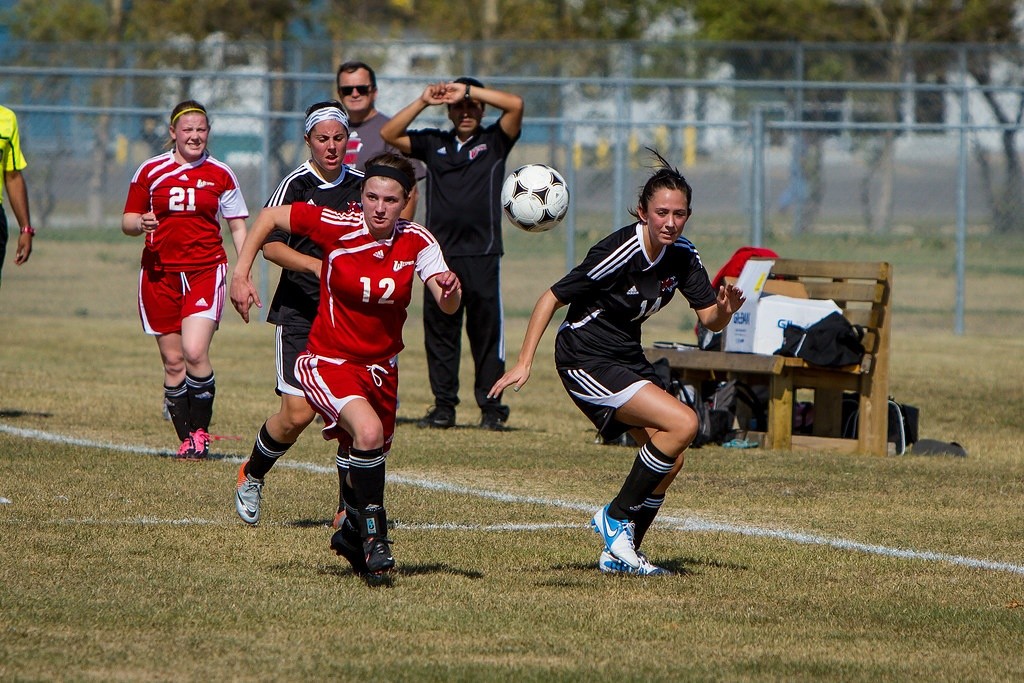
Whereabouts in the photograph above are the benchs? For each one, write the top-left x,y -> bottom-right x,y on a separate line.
641,253 -> 894,453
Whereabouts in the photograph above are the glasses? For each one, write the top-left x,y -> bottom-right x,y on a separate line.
340,84 -> 373,97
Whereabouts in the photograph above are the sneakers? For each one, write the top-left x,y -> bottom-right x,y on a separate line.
598,544 -> 671,576
479,411 -> 502,430
362,535 -> 395,571
417,407 -> 456,429
591,504 -> 640,569
234,460 -> 265,524
333,507 -> 347,528
176,438 -> 193,461
187,427 -> 211,461
330,529 -> 382,582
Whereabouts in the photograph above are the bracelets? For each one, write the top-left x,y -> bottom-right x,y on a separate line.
465,83 -> 470,98
19,226 -> 36,237
137,216 -> 145,233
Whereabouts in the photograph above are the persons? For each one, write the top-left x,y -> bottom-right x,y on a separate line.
0,105 -> 37,288
486,168 -> 746,574
237,102 -> 369,528
330,62 -> 427,222
228,154 -> 462,588
382,77 -> 526,428
121,101 -> 254,460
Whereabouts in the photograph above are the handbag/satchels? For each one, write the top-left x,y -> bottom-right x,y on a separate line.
773,310 -> 866,367
793,401 -> 816,435
607,378 -> 741,447
839,392 -> 919,456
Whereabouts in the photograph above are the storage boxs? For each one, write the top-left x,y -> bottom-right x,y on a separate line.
722,259 -> 843,355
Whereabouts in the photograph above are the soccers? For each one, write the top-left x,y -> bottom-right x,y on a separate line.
500,164 -> 570,233
